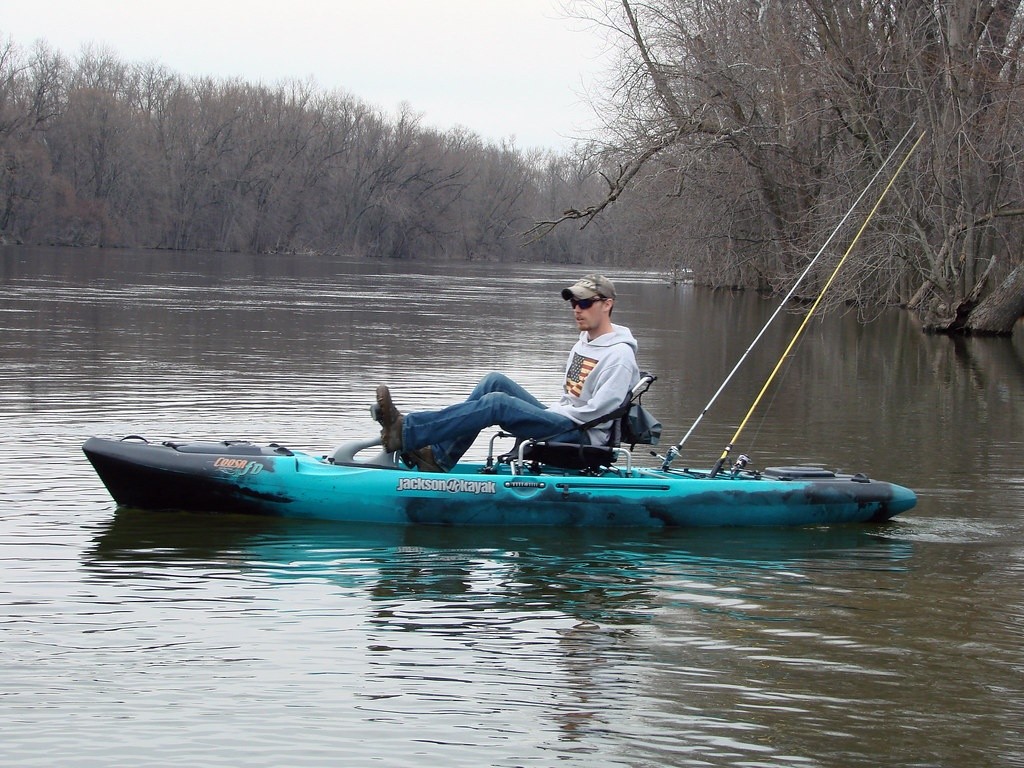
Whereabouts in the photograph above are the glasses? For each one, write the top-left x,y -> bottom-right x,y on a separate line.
571,298 -> 607,309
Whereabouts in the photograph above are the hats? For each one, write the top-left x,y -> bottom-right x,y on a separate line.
561,274 -> 616,301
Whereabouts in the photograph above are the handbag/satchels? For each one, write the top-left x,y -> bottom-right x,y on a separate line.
620,376 -> 662,452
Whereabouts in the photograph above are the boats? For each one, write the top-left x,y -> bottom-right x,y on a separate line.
80,433 -> 914,538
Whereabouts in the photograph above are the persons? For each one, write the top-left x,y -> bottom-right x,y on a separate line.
368,273 -> 642,473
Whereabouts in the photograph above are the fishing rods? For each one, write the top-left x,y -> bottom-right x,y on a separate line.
706,125 -> 932,480
659,120 -> 918,474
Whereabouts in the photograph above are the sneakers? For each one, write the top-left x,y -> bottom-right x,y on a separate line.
409,447 -> 443,472
377,386 -> 403,453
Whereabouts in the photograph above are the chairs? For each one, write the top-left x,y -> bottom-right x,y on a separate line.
483,369 -> 657,476
